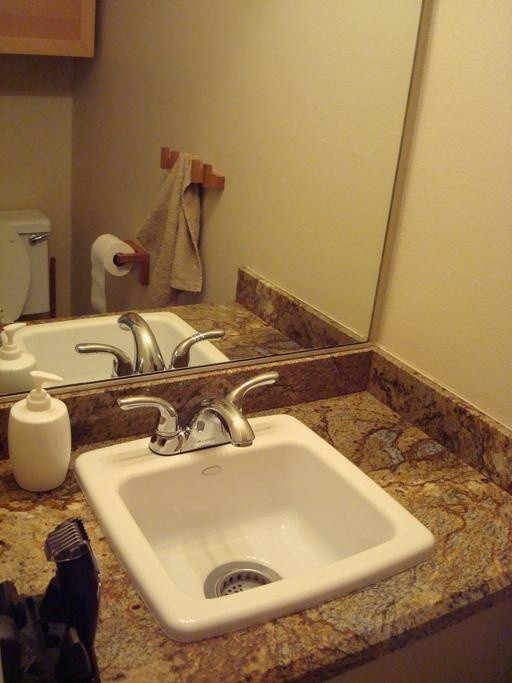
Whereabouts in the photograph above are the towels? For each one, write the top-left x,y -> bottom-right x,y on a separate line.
133,150 -> 204,309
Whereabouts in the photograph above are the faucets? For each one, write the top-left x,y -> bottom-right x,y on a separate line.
114,369 -> 280,454
74,311 -> 225,378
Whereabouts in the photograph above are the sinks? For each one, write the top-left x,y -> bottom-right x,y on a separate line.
71,411 -> 437,644
13,310 -> 230,386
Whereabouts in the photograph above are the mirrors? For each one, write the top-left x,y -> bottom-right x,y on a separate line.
1,0 -> 425,399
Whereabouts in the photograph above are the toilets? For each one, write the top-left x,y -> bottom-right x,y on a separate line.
0,207 -> 53,325
